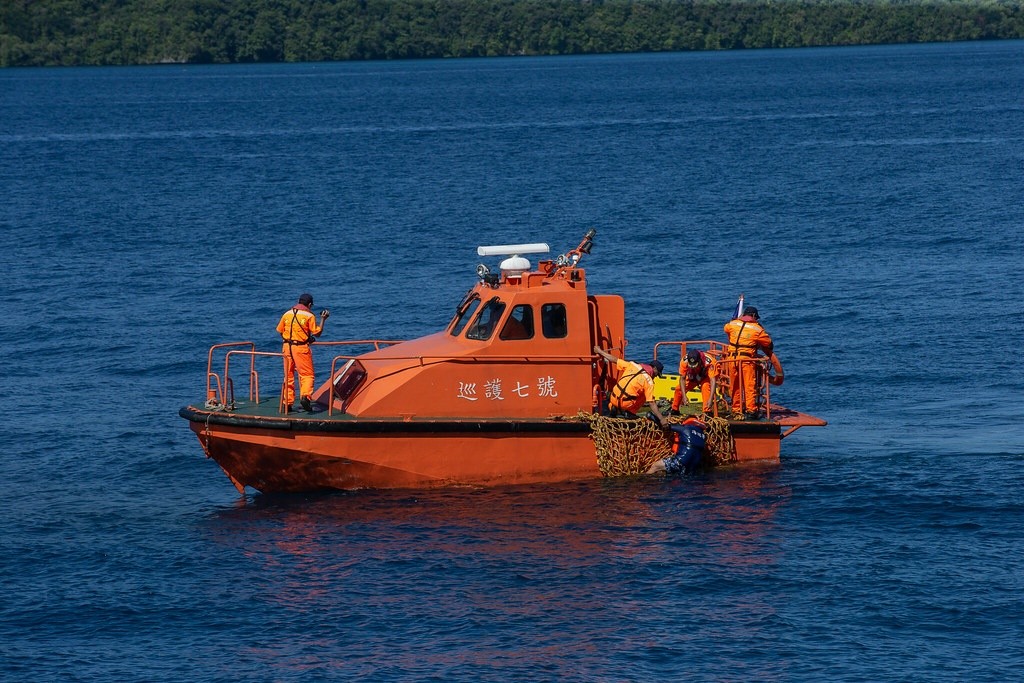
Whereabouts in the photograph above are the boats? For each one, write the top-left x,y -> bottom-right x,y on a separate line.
179,228 -> 829,492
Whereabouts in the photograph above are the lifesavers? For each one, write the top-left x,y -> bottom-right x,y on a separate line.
760,353 -> 783,386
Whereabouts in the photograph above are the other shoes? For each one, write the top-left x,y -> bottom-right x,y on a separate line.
608,406 -> 617,419
705,412 -> 714,420
670,409 -> 680,416
748,412 -> 762,420
283,405 -> 292,411
300,397 -> 314,414
624,410 -> 641,420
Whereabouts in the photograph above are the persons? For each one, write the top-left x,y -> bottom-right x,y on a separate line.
276,294 -> 331,415
724,307 -> 773,420
593,345 -> 669,434
646,416 -> 708,476
661,349 -> 721,422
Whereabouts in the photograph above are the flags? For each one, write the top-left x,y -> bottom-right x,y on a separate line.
730,300 -> 741,320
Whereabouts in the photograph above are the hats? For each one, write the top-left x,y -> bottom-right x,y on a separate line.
299,294 -> 315,306
687,350 -> 700,369
744,307 -> 760,319
652,360 -> 663,378
